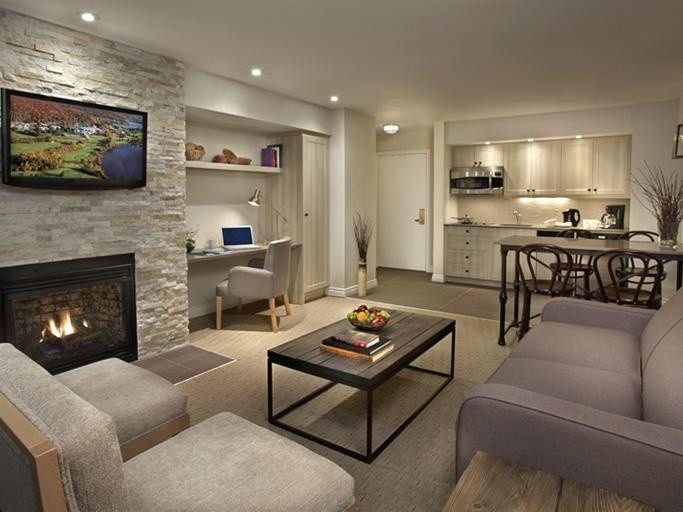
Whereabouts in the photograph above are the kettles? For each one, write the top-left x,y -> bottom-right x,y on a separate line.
561,209 -> 580,226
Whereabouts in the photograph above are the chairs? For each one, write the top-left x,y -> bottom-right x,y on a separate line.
546,227 -> 595,300
0,341 -> 360,510
513,243 -> 573,345
589,249 -> 668,310
214,235 -> 297,333
612,230 -> 666,291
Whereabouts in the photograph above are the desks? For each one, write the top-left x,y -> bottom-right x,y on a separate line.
188,239 -> 301,328
493,235 -> 682,346
438,449 -> 656,512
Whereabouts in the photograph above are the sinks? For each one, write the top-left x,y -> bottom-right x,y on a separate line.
488,223 -> 533,227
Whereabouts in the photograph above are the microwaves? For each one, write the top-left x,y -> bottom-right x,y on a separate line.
448,166 -> 503,195
460,222 -> 496,225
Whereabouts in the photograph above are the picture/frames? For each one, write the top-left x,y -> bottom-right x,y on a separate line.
674,124 -> 682,158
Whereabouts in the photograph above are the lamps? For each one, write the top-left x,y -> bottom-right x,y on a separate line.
247,189 -> 291,245
382,124 -> 399,135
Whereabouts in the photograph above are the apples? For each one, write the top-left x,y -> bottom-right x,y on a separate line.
368,306 -> 381,313
348,312 -> 357,321
372,316 -> 386,325
357,305 -> 367,312
380,310 -> 389,318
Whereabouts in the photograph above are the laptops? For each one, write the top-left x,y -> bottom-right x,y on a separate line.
221,225 -> 260,249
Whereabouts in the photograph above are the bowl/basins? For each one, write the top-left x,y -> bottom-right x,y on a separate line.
348,316 -> 388,332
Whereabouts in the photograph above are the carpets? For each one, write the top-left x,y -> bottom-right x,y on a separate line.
130,343 -> 236,386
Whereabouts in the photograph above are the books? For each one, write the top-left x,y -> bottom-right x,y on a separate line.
261,143 -> 283,168
319,329 -> 395,362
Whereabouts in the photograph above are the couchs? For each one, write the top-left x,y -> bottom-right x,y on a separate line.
454,283 -> 682,512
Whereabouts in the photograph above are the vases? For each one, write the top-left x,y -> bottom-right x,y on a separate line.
356,257 -> 368,297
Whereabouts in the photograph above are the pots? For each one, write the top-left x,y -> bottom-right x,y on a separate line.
451,214 -> 472,223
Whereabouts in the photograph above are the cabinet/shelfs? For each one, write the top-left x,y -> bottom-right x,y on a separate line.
490,226 -> 536,288
560,134 -> 630,197
503,140 -> 560,199
450,144 -> 502,168
442,225 -> 490,286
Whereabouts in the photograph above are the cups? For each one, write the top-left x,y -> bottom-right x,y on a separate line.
209,239 -> 217,249
583,220 -> 600,229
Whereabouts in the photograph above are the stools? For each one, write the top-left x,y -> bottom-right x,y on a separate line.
53,357 -> 190,463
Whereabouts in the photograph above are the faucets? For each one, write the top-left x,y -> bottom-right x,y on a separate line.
513,210 -> 521,224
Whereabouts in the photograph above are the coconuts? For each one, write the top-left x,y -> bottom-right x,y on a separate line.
195,146 -> 204,152
186,142 -> 196,155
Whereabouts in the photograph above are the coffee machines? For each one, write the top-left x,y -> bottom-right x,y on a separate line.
601,205 -> 625,229
